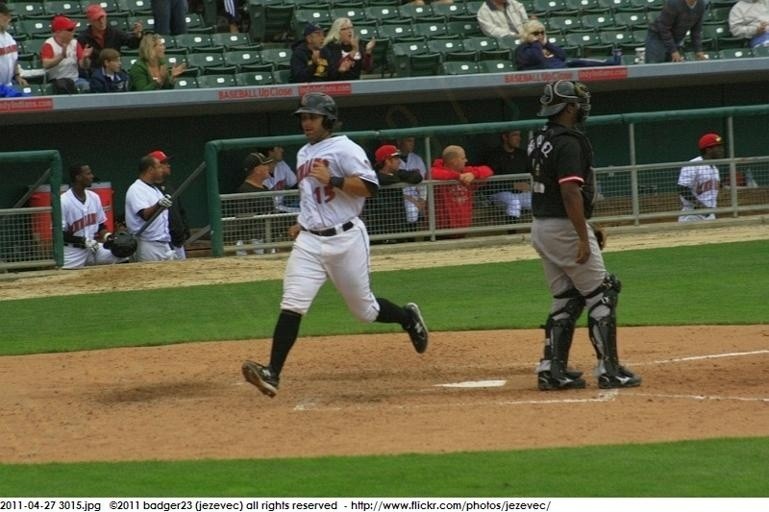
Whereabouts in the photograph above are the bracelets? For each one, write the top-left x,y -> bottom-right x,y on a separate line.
329,176 -> 344,190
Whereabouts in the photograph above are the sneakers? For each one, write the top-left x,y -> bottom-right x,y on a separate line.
538,365 -> 587,391
597,367 -> 642,389
242,359 -> 279,398
403,302 -> 429,354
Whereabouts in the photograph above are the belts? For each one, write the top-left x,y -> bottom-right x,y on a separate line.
300,221 -> 354,237
64,243 -> 81,248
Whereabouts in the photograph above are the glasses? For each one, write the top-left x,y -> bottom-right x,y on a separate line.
531,31 -> 544,36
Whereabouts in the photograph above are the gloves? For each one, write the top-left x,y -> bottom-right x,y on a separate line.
81,238 -> 100,254
158,194 -> 177,209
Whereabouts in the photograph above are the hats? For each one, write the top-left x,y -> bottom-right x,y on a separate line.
697,132 -> 725,152
148,149 -> 176,164
52,15 -> 77,34
303,24 -> 321,35
243,152 -> 275,171
86,4 -> 108,21
374,144 -> 404,163
0,4 -> 16,14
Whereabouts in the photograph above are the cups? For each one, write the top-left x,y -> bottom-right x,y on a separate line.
612,48 -> 624,66
635,47 -> 647,64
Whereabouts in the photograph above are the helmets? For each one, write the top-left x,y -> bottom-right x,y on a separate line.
103,233 -> 139,257
295,91 -> 338,121
537,79 -> 580,117
573,81 -> 592,121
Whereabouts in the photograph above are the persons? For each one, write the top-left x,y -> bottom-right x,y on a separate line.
323,16 -> 376,80
0,1 -> 248,99
263,145 -> 301,228
518,20 -> 621,66
59,150 -> 186,268
528,79 -> 642,389
476,1 -> 527,48
366,128 -> 535,247
290,26 -> 333,81
728,1 -> 769,58
644,1 -> 707,61
241,92 -> 428,399
235,151 -> 277,257
677,131 -> 724,222
